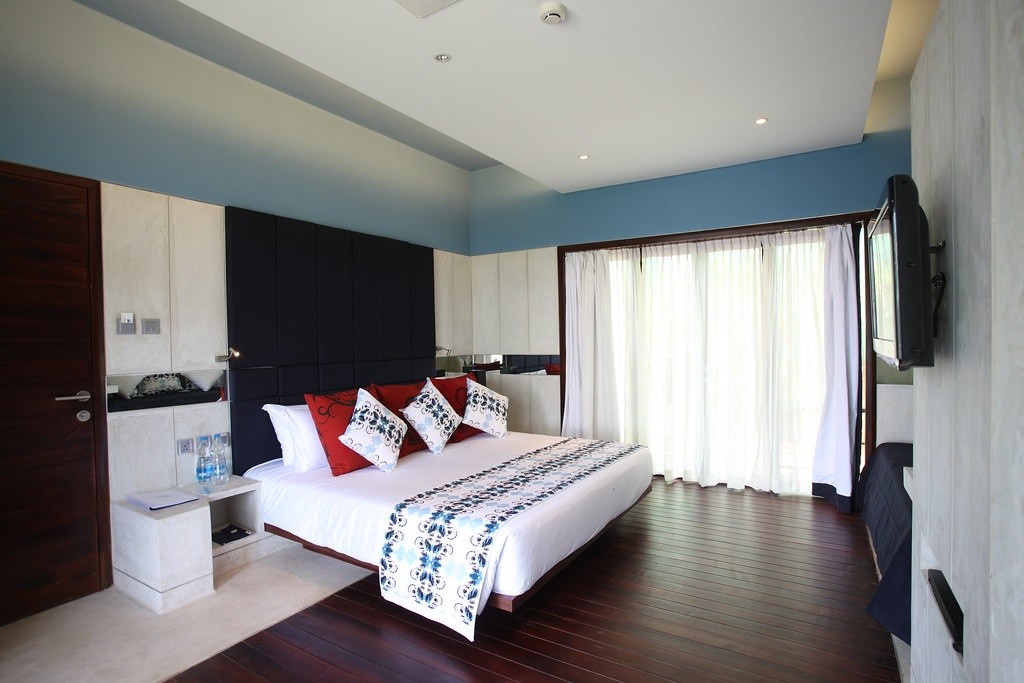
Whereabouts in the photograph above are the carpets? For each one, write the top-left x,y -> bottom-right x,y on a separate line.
0,536 -> 375,683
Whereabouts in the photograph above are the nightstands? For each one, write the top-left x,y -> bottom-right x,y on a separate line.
112,474 -> 266,617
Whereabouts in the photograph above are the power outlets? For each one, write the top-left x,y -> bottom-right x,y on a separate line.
178,438 -> 193,456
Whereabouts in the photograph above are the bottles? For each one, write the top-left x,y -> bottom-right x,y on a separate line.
196,434 -> 229,488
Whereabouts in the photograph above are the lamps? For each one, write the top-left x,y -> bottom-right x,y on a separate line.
436,346 -> 451,356
216,347 -> 239,362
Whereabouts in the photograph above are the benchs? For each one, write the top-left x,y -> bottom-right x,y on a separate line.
866,384 -> 913,648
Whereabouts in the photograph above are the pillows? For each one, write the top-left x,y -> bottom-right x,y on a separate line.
262,403 -> 310,468
283,408 -> 330,473
338,388 -> 407,473
304,384 -> 407,477
374,380 -> 453,459
398,377 -> 463,454
431,372 -> 484,443
461,378 -> 510,438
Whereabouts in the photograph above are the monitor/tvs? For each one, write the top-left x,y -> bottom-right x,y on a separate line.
864,175 -> 936,372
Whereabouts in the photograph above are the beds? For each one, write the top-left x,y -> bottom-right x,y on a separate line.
242,430 -> 652,642
